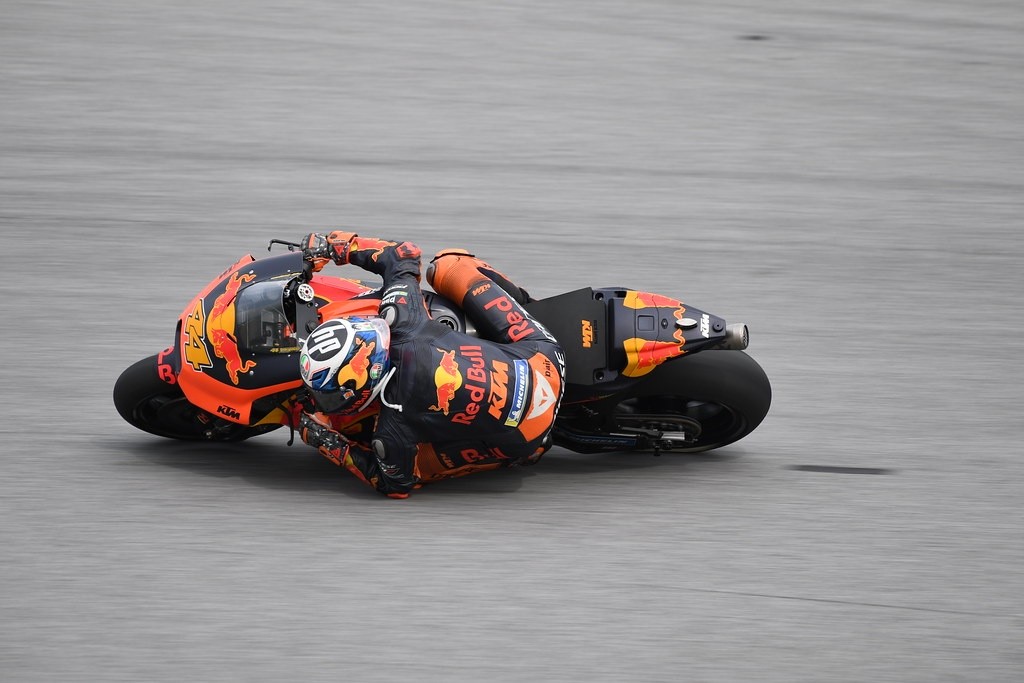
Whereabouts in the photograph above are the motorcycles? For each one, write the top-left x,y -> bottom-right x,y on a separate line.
111,238 -> 774,460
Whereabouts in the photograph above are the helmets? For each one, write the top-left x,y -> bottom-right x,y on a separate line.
298,316 -> 391,416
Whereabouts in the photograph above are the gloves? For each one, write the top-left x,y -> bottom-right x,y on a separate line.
299,413 -> 351,467
301,230 -> 358,265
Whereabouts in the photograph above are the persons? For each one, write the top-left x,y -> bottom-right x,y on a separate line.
297,232 -> 567,501
